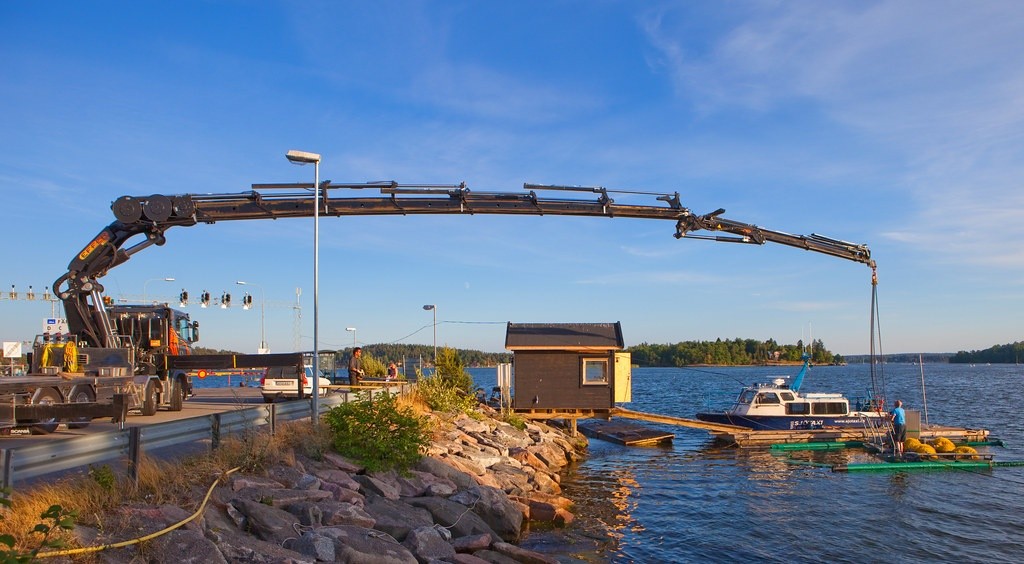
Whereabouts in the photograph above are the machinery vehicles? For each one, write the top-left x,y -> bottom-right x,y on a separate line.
0,180 -> 877,436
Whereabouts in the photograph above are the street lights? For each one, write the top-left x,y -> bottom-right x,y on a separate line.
143,278 -> 175,306
346,328 -> 356,356
237,281 -> 266,351
285,144 -> 323,429
421,304 -> 438,378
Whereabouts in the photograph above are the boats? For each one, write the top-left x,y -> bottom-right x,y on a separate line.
696,354 -> 893,431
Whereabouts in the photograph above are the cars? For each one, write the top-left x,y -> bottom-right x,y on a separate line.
259,363 -> 332,404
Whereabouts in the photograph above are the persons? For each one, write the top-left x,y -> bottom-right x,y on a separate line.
389,362 -> 398,379
856,401 -> 861,411
348,347 -> 365,393
888,400 -> 907,452
